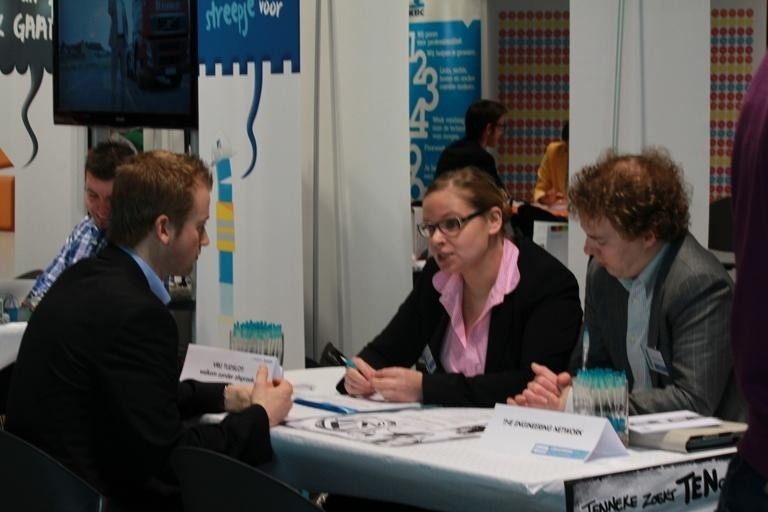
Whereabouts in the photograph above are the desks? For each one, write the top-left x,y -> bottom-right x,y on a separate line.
200,407 -> 739,512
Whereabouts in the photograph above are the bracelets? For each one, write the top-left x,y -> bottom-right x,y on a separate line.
221,385 -> 230,413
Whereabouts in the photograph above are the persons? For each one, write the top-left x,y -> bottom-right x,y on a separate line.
533,120 -> 568,206
344,167 -> 583,406
718,48 -> 768,510
138,3 -> 156,79
10,149 -> 293,511
21,132 -> 137,311
106,2 -> 128,114
505,146 -> 749,424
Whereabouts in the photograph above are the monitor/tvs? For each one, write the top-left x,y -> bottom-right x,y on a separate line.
53,0 -> 199,130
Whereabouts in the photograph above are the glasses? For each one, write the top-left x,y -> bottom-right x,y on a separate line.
417,212 -> 486,238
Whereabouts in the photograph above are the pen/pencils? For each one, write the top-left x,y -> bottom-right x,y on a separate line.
457,425 -> 485,433
339,355 -> 357,368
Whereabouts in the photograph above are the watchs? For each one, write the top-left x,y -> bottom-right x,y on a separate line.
434,99 -> 510,242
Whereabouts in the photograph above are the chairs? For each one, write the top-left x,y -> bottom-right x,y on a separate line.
144,445 -> 321,511
710,197 -> 733,251
517,205 -> 567,237
0,428 -> 103,512
